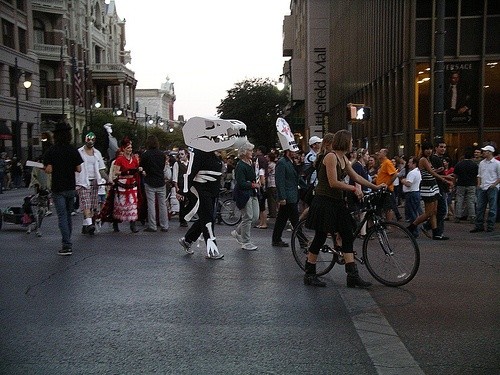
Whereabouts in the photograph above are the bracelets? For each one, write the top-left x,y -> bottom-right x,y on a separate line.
252,183 -> 254,188
353,186 -> 356,192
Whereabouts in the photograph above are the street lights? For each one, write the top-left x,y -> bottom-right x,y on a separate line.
155,111 -> 165,128
165,120 -> 174,133
13,56 -> 34,157
89,88 -> 104,132
144,108 -> 154,138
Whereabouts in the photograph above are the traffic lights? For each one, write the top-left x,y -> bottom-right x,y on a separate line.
356,105 -> 374,120
348,105 -> 357,120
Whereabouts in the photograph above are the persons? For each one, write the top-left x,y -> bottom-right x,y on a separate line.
266,152 -> 277,218
342,140 -> 484,240
254,146 -> 268,228
74,130 -> 115,236
444,71 -> 473,123
178,127 -> 225,259
303,130 -> 388,288
139,134 -> 169,232
470,145 -> 500,233
0,151 -> 32,194
290,135 -> 324,230
108,149 -> 124,189
28,156 -> 53,217
97,177 -> 107,213
42,122 -> 85,256
272,148 -> 311,249
71,188 -> 80,216
231,142 -> 260,250
172,148 -> 199,227
112,137 -> 140,232
313,133 -> 335,178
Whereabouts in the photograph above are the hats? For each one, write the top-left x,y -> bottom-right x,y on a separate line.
308,135 -> 324,146
481,145 -> 495,152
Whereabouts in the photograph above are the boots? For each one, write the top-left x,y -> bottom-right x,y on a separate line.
113,222 -> 121,232
344,262 -> 373,288
304,260 -> 326,287
130,221 -> 140,233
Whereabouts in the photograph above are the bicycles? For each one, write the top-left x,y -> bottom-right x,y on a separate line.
215,188 -> 242,226
290,187 -> 421,288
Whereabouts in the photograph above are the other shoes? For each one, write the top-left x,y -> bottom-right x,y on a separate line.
432,230 -> 450,241
81,223 -> 96,235
178,237 -> 194,254
231,230 -> 242,244
205,252 -> 224,259
57,247 -> 72,255
253,224 -> 268,229
143,227 -> 157,232
161,228 -> 168,232
406,226 -> 418,238
272,240 -> 289,247
469,225 -> 493,234
241,243 -> 258,250
